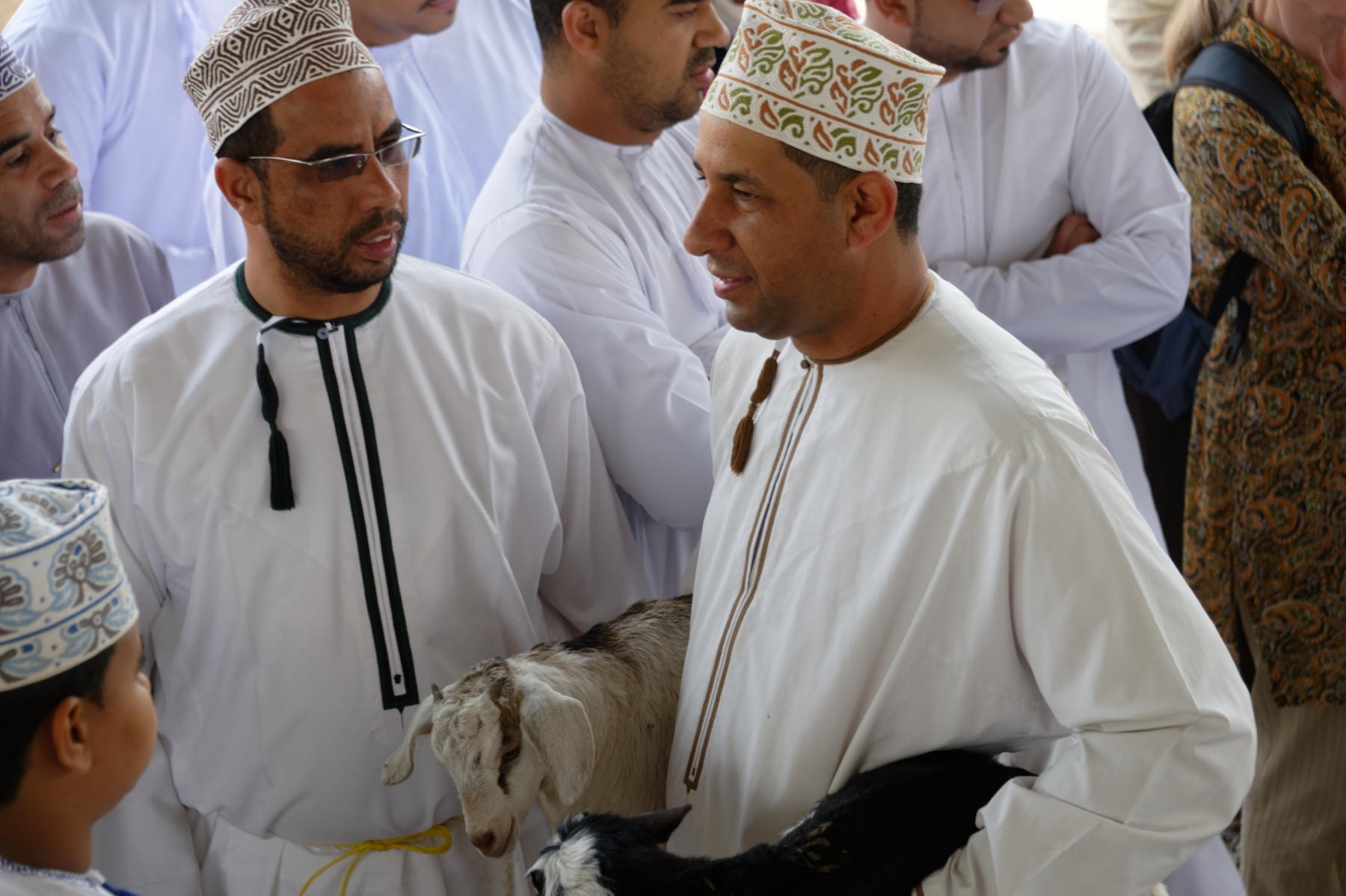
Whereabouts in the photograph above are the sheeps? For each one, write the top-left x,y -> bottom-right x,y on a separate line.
521,748 -> 1032,896
386,593 -> 701,861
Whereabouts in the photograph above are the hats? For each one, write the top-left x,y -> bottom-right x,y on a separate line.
0,478 -> 140,692
1,35 -> 36,100
700,0 -> 945,184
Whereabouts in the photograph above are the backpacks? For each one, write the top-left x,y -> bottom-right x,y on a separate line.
1114,39 -> 1312,427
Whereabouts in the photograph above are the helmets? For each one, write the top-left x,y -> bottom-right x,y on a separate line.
181,0 -> 381,156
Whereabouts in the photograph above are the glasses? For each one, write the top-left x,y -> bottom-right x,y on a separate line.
242,123 -> 425,183
971,0 -> 1004,16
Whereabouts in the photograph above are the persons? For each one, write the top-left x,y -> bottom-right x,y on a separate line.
860,0 -> 1244,896
661,0 -> 1257,896
0,478 -> 156,896
1104,0 -> 1346,896
465,0 -> 730,598
61,0 -> 647,896
0,0 -> 541,298
0,40 -> 176,485
